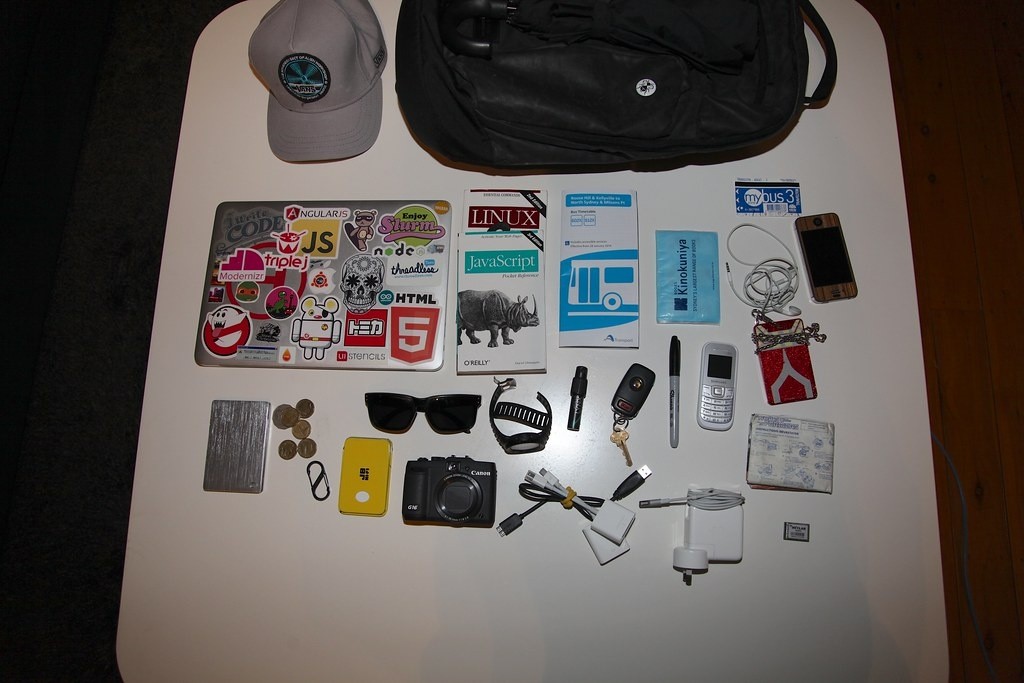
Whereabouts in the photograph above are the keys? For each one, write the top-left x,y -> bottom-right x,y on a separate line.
611,364 -> 656,466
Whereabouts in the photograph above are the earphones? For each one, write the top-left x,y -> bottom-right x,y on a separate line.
773,305 -> 802,315
776,266 -> 798,285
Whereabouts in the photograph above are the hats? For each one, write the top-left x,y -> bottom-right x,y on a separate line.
248,0 -> 389,163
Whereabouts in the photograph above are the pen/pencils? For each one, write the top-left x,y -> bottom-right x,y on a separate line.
669,335 -> 681,448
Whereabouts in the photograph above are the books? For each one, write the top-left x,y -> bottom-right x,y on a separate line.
558,191 -> 639,348
457,190 -> 546,374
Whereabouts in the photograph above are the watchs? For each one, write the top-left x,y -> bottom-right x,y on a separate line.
488,376 -> 552,455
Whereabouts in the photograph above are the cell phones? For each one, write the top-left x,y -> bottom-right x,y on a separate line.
696,342 -> 739,431
794,213 -> 858,303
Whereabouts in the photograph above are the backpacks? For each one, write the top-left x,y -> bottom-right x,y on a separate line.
395,0 -> 835,176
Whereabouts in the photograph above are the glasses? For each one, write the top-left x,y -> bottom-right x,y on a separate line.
364,392 -> 482,436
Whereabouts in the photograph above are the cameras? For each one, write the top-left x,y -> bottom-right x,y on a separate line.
402,455 -> 497,528
